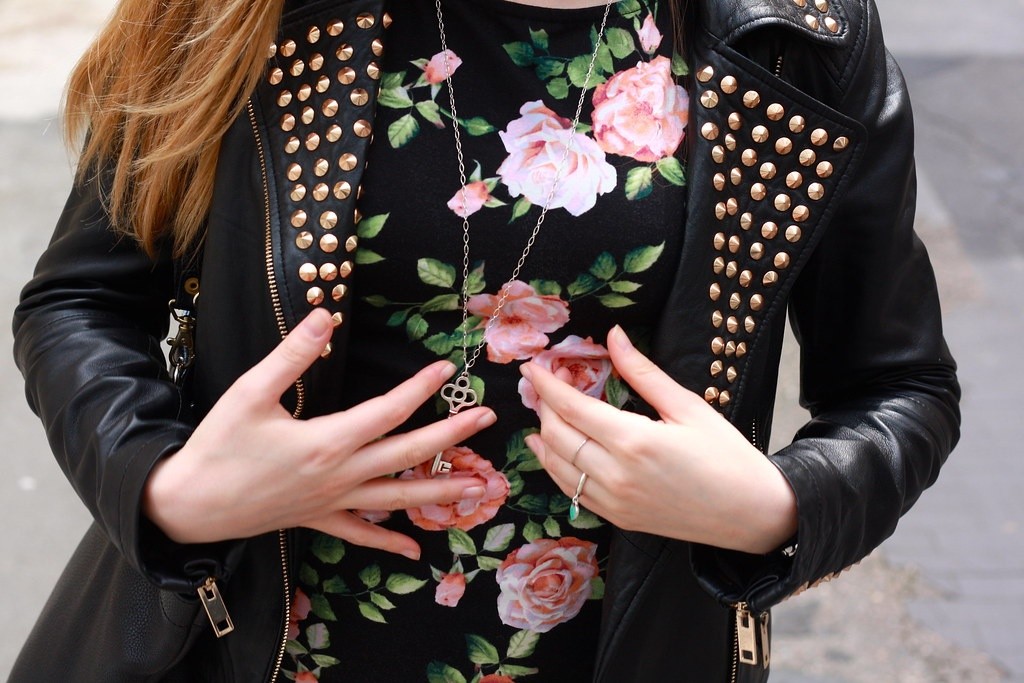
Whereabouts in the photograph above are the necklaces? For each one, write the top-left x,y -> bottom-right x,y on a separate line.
429,0 -> 612,479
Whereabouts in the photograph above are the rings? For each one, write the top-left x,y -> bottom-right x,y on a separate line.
569,471 -> 589,522
571,436 -> 590,466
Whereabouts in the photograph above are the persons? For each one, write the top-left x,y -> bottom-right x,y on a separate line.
6,0 -> 963,683
